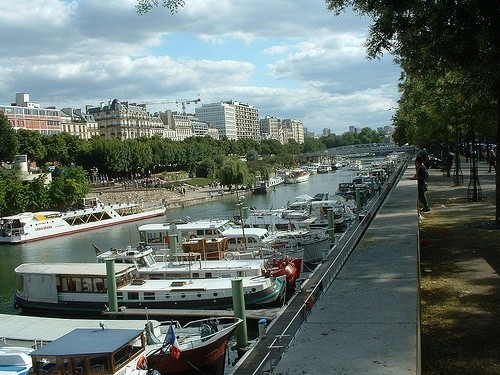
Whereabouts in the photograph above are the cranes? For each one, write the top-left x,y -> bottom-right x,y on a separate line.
139,94 -> 201,112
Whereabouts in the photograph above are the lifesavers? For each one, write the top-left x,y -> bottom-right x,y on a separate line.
237,244 -> 246,253
136,356 -> 148,370
224,252 -> 236,262
365,190 -> 369,196
190,234 -> 198,238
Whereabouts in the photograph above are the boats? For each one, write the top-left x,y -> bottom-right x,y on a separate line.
348,159 -> 362,170
363,151 -> 408,191
1,199 -> 333,375
251,159 -> 349,193
282,175 -> 380,234
0,196 -> 166,244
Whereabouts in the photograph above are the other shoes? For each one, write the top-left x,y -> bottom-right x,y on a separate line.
420,208 -> 425,212
424,208 -> 429,212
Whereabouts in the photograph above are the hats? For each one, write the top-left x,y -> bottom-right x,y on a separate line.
415,156 -> 422,162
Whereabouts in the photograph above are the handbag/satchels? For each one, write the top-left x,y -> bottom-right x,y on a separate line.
423,183 -> 427,191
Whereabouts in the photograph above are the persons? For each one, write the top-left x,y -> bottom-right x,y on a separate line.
408,156 -> 431,213
416,148 -> 428,160
488,150 -> 496,173
446,153 -> 454,178
